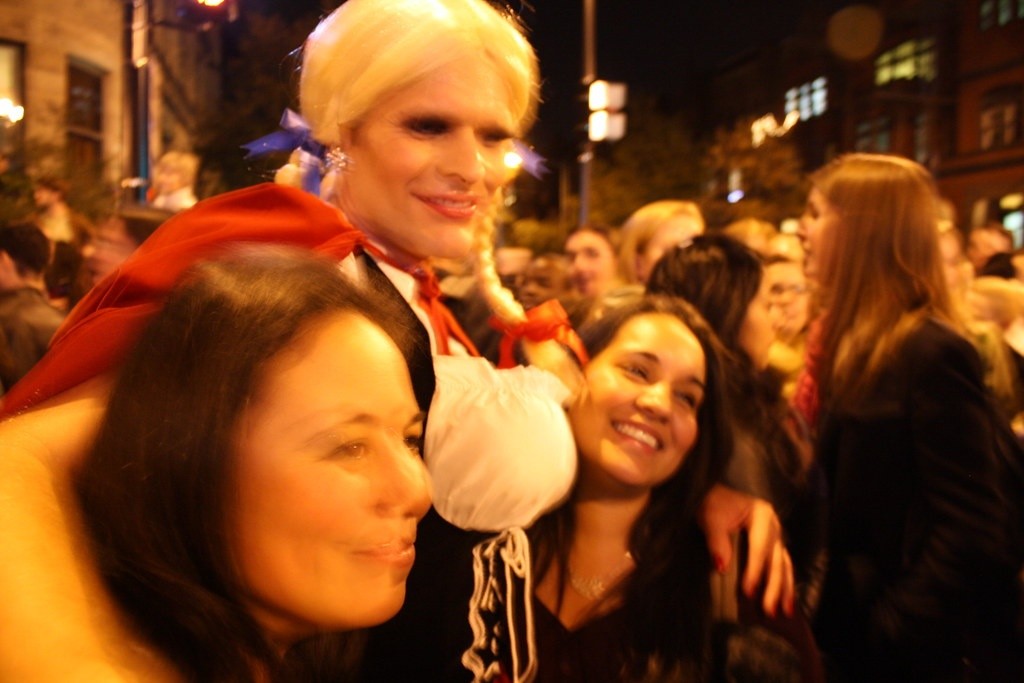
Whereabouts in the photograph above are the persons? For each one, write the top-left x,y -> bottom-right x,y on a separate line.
82,250 -> 433,683
793,154 -> 1024,683
936,224 -> 1024,433
0,0 -> 795,683
0,175 -> 141,393
642,232 -> 823,683
534,294 -> 727,683
154,126 -> 194,210
496,199 -> 813,401
